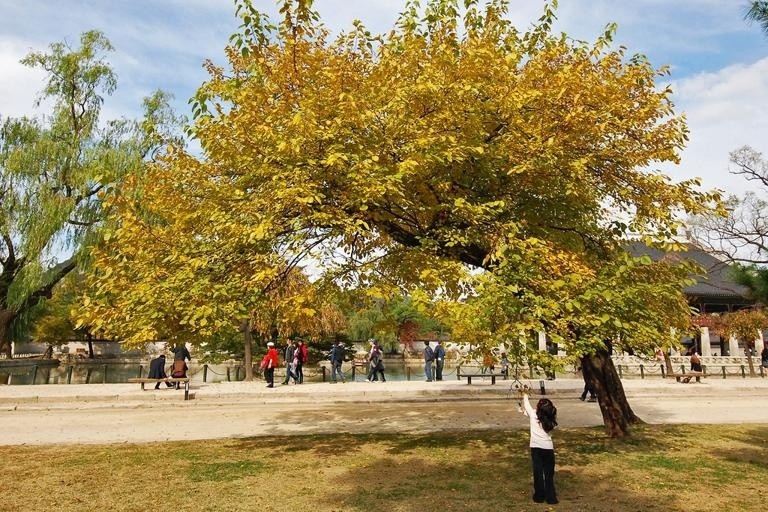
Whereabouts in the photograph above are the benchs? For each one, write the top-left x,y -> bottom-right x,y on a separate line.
666,374 -> 710,382
128,378 -> 192,390
457,374 -> 506,384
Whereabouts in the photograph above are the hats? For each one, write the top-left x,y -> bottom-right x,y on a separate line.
267,342 -> 274,346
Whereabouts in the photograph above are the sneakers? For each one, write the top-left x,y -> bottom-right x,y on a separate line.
364,378 -> 386,383
266,384 -> 273,387
281,381 -> 288,384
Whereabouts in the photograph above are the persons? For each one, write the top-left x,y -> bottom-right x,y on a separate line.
744,347 -> 757,356
149,355 -> 172,389
434,339 -> 445,381
501,353 -> 508,380
262,342 -> 276,387
761,343 -> 768,368
47,347 -> 51,359
172,339 -> 191,389
331,338 -> 386,384
523,392 -> 559,504
690,351 -> 702,383
281,337 -> 303,384
579,384 -> 596,402
424,341 -> 434,382
